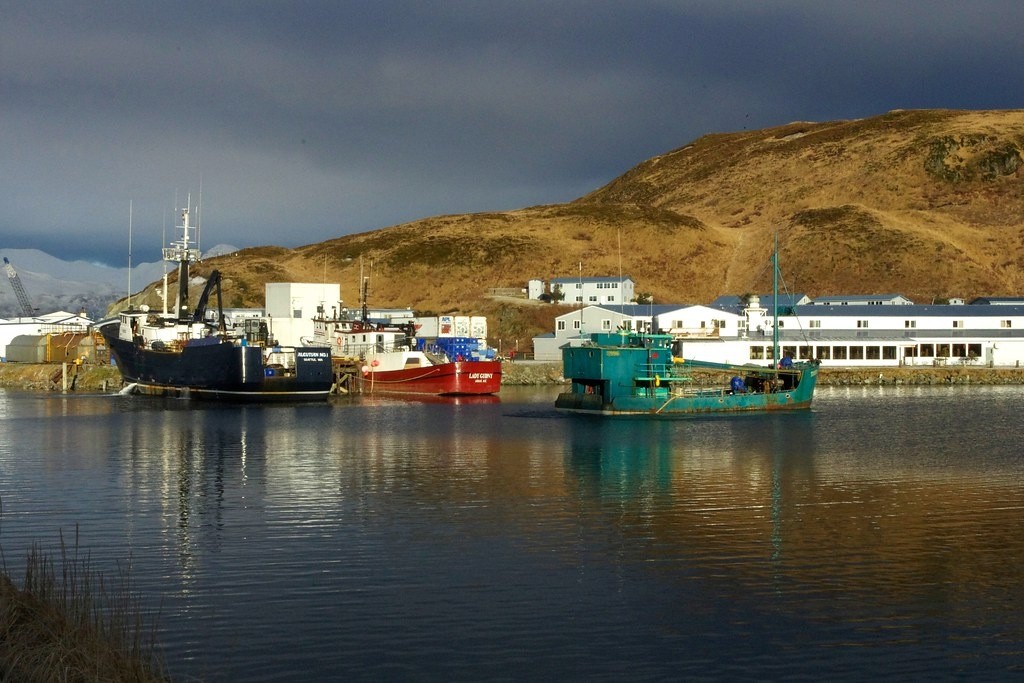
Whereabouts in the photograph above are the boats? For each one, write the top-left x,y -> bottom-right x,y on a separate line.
554,229 -> 822,420
299,274 -> 502,397
98,173 -> 332,403
